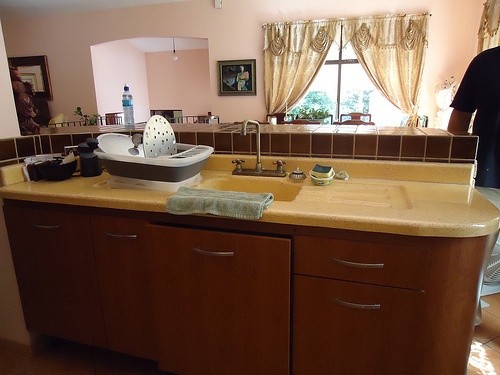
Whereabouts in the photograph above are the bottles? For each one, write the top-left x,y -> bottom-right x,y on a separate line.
122,86 -> 134,126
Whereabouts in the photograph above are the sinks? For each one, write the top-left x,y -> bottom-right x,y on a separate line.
194,176 -> 304,203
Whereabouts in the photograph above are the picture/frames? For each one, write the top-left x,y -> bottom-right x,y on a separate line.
216,59 -> 257,96
7,55 -> 54,101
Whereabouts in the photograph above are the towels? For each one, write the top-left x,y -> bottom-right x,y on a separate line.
165,186 -> 274,220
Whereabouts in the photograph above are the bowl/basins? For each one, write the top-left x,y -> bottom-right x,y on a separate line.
309,170 -> 335,185
36,160 -> 77,181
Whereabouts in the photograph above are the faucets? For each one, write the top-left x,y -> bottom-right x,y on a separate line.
242,119 -> 262,176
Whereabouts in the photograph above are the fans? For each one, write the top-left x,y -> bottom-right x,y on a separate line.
474,187 -> 500,296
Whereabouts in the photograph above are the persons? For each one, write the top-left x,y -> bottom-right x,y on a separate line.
448,47 -> 500,189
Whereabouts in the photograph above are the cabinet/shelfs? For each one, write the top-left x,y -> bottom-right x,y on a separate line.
3,199 -> 500,375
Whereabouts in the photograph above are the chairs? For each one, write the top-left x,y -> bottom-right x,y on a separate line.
106,111 -> 125,125
266,112 -> 375,125
406,115 -> 429,128
